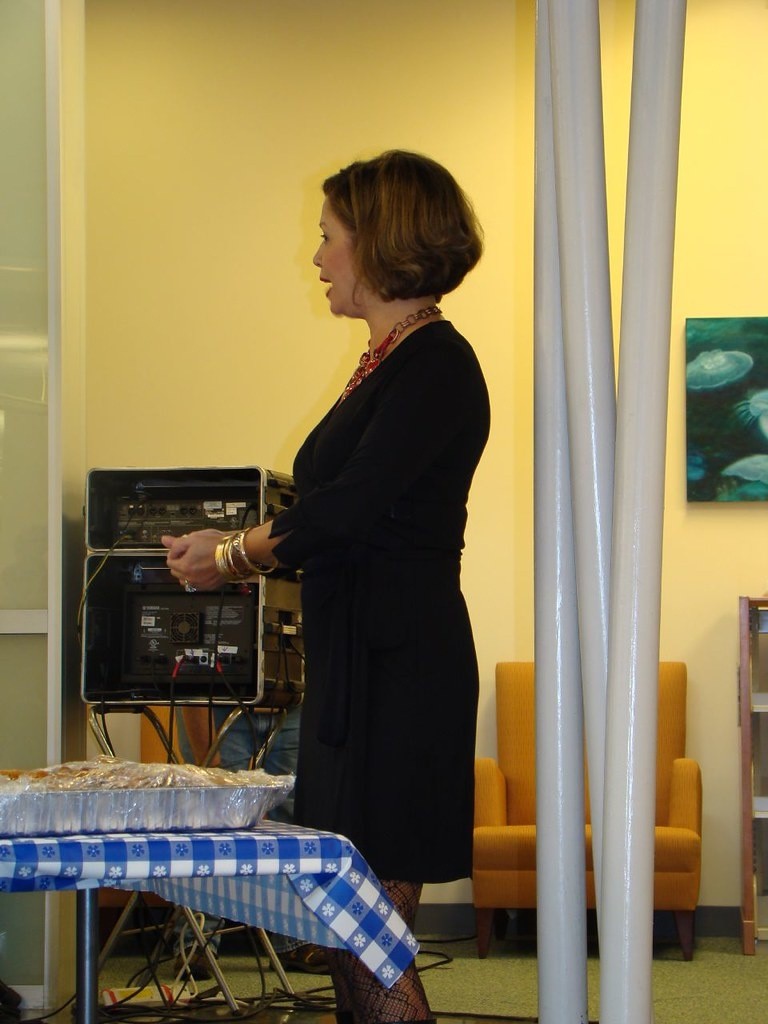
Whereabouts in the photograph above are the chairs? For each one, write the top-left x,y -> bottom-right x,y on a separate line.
473,661 -> 702,958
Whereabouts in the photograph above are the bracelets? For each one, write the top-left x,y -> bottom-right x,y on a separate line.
215,525 -> 279,583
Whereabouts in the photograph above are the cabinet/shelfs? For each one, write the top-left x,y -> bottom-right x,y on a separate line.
738,596 -> 768,955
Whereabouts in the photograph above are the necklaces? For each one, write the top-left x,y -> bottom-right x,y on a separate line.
340,306 -> 443,402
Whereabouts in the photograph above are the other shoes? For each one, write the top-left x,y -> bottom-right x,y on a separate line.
270,943 -> 330,974
174,946 -> 215,980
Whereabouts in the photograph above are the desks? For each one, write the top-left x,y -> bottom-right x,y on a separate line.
0,817 -> 419,1024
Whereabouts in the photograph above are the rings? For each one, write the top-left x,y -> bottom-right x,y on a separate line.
182,534 -> 188,538
184,579 -> 199,593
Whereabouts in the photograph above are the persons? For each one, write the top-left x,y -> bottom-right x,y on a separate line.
161,149 -> 491,1024
173,704 -> 332,981
